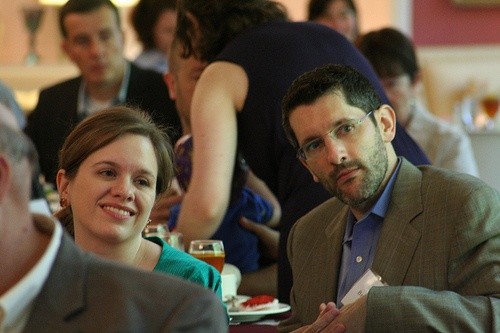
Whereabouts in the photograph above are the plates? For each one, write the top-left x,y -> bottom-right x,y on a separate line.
228,303 -> 291,322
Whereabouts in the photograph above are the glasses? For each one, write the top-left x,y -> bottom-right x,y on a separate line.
296,111 -> 375,161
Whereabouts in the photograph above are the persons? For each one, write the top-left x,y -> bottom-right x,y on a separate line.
273,64 -> 500,333
307,0 -> 365,49
0,123 -> 231,333
52,107 -> 230,326
357,28 -> 477,176
171,0 -> 433,305
0,0 -> 282,276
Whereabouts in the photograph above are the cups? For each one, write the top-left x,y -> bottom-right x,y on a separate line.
144,224 -> 184,252
189,240 -> 224,273
